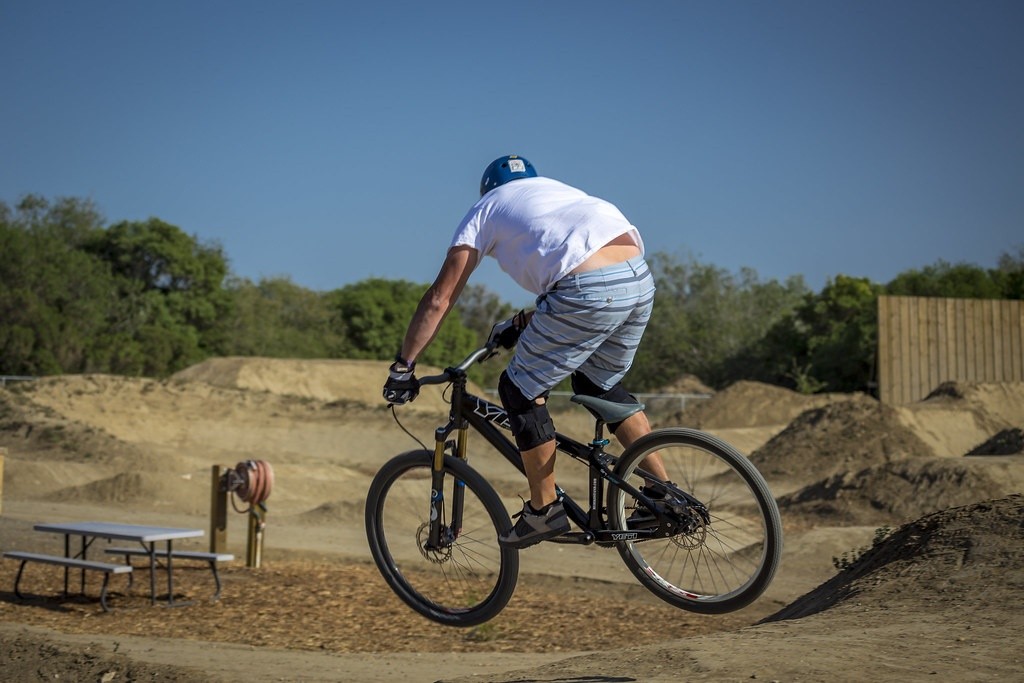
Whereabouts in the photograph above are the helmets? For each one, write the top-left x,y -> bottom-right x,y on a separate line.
478,154 -> 540,196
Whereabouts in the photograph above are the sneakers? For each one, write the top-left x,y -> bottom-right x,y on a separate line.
622,483 -> 683,530
497,495 -> 572,550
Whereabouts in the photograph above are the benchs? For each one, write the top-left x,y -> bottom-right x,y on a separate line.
106,546 -> 233,603
4,551 -> 134,612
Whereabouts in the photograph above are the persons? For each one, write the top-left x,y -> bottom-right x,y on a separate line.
383,155 -> 685,549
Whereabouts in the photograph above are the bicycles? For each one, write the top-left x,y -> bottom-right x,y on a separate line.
365,334 -> 782,630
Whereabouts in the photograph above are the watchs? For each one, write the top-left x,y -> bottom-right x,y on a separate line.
395,352 -> 416,368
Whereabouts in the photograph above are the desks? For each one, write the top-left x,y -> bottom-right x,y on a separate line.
32,523 -> 204,606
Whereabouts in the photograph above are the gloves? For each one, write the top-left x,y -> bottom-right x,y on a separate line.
382,361 -> 421,406
477,309 -> 532,364
